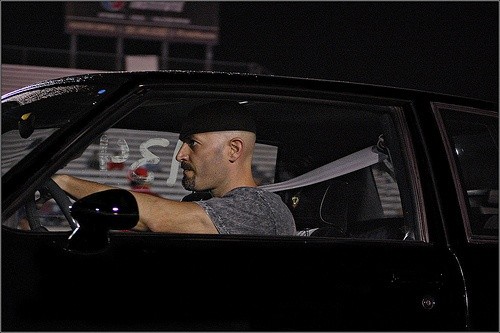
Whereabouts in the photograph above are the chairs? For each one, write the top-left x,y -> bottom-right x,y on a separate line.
280,142 -> 407,237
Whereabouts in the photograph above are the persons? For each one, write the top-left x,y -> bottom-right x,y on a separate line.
36,100 -> 297,236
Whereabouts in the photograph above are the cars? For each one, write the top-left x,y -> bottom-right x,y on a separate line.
1,68 -> 499,333
